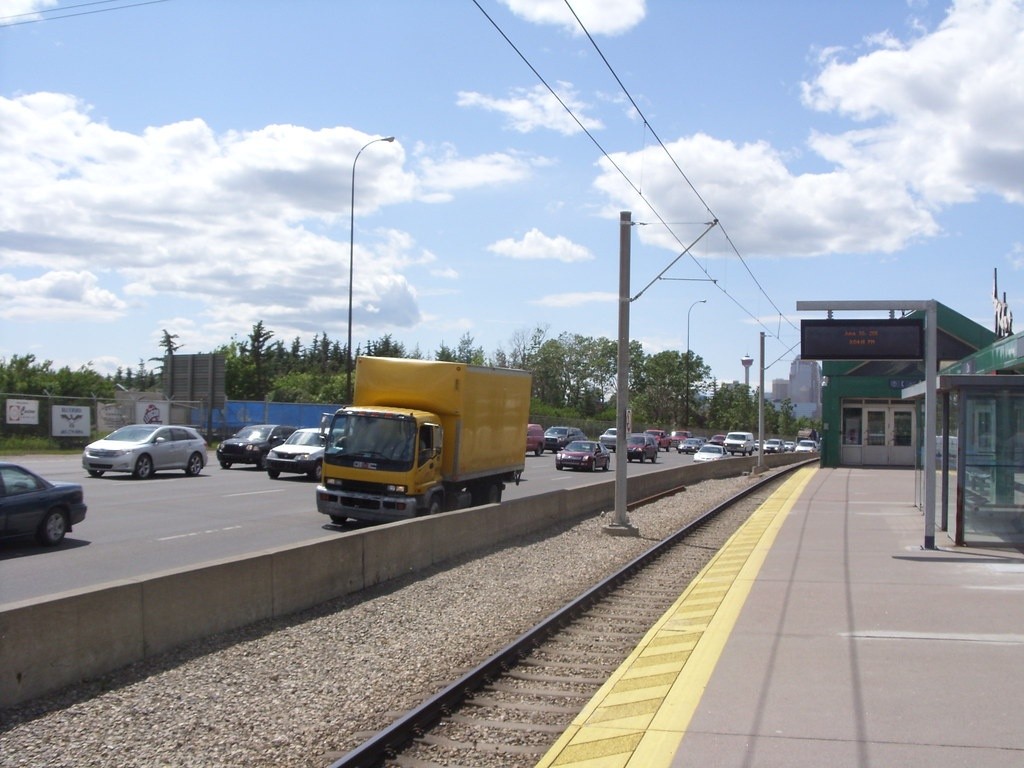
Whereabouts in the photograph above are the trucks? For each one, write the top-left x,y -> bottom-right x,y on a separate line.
316,354 -> 533,523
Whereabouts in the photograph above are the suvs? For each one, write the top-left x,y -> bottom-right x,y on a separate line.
266,428 -> 346,480
81,424 -> 209,478
544,426 -> 591,453
216,423 -> 300,472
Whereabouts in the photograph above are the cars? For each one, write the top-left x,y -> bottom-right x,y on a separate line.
555,440 -> 612,473
0,462 -> 88,546
599,428 -> 821,467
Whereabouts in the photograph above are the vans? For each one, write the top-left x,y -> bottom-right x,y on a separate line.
525,423 -> 545,456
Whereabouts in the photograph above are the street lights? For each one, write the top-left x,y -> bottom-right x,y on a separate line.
343,133 -> 397,404
685,299 -> 707,429
